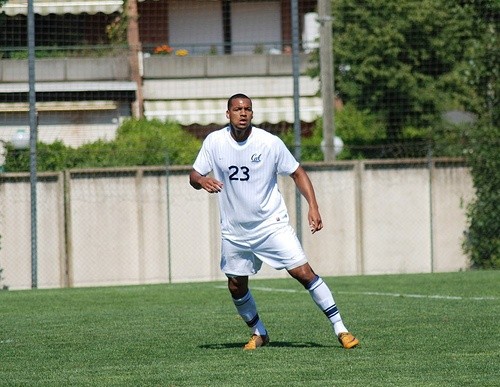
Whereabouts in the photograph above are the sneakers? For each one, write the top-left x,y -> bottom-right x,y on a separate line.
244,329 -> 269,350
338,332 -> 358,349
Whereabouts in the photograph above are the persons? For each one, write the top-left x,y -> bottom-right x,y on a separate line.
189,93 -> 359,350
